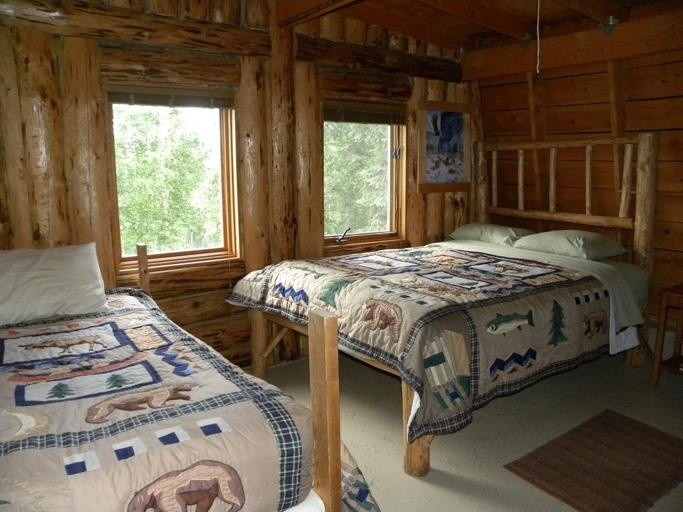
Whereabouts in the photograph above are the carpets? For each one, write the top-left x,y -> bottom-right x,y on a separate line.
501,410 -> 681,512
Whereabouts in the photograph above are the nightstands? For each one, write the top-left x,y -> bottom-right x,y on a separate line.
651,283 -> 682,390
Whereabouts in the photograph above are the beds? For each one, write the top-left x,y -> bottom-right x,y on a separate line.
0,244 -> 379,512
225,131 -> 659,482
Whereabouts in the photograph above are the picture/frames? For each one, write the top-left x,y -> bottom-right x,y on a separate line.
416,100 -> 476,194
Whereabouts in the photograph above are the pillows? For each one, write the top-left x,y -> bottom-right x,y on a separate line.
513,229 -> 629,261
0,241 -> 110,327
447,222 -> 535,245
